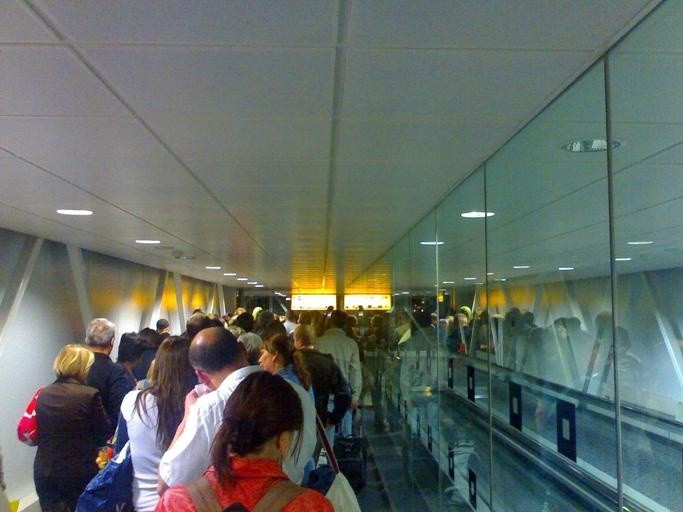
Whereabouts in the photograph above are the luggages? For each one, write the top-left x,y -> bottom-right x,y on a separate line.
330,403 -> 367,495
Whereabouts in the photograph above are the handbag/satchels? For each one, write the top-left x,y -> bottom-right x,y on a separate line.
14,384 -> 48,448
72,434 -> 138,512
299,467 -> 362,512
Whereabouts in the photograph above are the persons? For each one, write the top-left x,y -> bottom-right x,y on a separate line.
33,306 -> 369,512
396,300 -> 664,474
